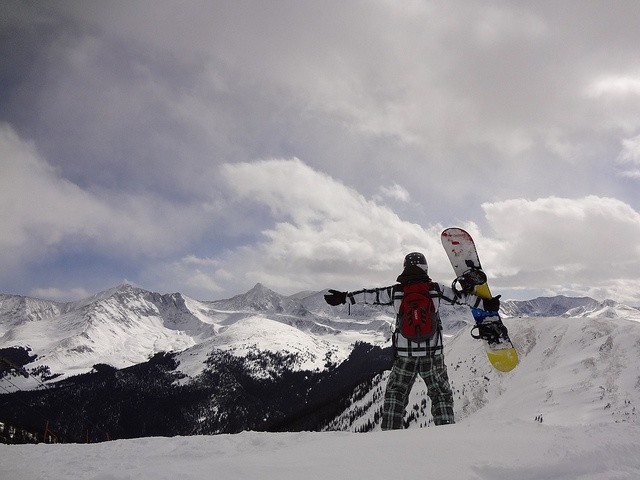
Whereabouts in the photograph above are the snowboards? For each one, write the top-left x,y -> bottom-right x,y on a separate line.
440,228 -> 518,372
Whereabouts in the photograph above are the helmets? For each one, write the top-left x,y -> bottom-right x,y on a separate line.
402,251 -> 428,272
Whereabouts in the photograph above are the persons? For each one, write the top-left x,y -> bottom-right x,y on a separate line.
324,252 -> 501,431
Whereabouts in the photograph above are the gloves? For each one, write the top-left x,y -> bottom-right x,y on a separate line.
482,294 -> 502,312
324,289 -> 346,307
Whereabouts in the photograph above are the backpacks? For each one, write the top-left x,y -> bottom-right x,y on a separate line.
397,279 -> 439,342
